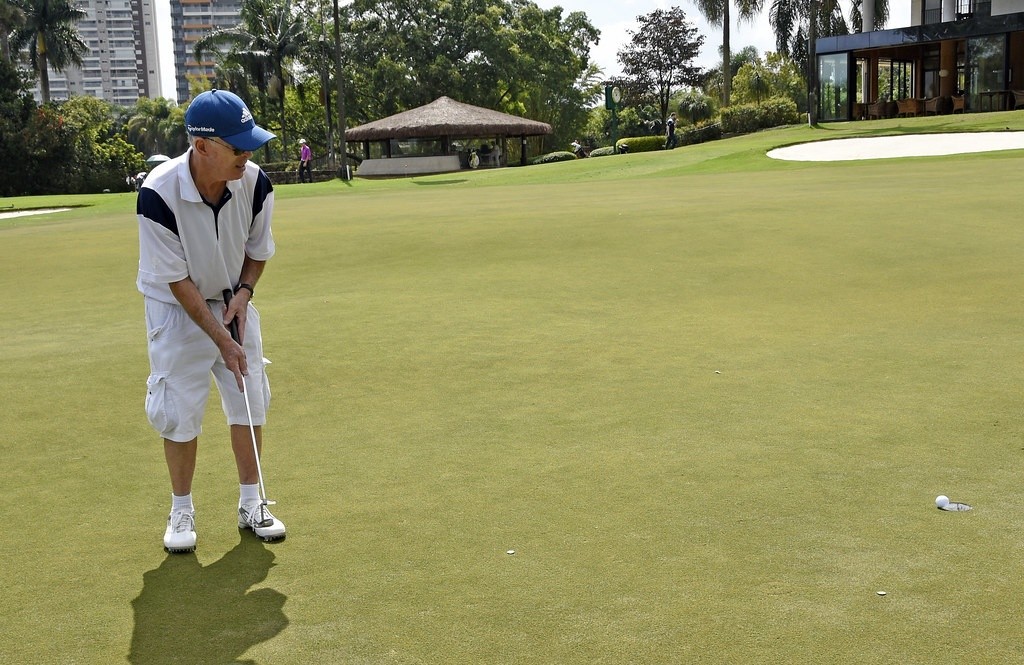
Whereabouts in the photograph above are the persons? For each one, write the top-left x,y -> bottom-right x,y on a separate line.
665,112 -> 678,150
572,140 -> 590,159
643,120 -> 661,136
488,141 -> 501,167
136,89 -> 285,552
298,139 -> 312,184
126,174 -> 148,192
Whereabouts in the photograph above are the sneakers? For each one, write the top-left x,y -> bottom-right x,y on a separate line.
163,510 -> 197,553
237,498 -> 286,541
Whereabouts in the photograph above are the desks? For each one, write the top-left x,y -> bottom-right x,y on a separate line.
980,91 -> 1011,111
856,103 -> 873,120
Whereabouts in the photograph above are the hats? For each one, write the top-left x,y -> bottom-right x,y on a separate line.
184,88 -> 278,151
299,138 -> 306,144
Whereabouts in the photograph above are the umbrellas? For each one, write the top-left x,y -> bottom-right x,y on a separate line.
146,154 -> 170,165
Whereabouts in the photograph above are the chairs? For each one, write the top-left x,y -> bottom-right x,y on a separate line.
951,94 -> 965,113
868,95 -> 944,120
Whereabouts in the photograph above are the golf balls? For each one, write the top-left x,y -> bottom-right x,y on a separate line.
935,494 -> 949,508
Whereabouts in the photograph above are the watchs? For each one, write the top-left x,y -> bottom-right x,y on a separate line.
233,283 -> 255,302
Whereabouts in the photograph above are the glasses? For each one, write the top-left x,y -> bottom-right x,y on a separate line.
200,137 -> 246,156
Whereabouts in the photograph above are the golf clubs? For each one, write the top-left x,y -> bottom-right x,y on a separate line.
222,288 -> 277,529
